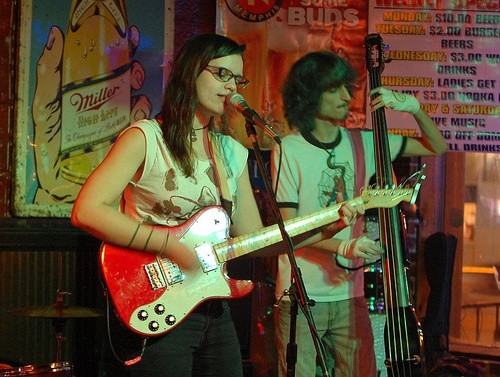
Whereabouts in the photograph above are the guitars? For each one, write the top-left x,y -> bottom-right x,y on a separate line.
100,165 -> 427,337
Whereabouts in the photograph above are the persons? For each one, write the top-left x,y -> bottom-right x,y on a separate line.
270,51 -> 448,377
71,34 -> 365,377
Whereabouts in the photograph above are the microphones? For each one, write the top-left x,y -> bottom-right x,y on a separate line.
225,92 -> 281,142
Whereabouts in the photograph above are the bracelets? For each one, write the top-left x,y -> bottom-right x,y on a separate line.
143,226 -> 154,251
127,224 -> 140,248
159,228 -> 169,255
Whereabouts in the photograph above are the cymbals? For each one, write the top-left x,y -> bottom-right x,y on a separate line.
7,306 -> 104,318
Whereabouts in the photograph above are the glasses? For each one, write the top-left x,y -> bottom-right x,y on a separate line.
207,65 -> 251,89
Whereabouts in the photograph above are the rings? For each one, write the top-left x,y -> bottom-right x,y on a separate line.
350,214 -> 357,222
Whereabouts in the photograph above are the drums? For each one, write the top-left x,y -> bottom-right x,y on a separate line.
0,360 -> 74,377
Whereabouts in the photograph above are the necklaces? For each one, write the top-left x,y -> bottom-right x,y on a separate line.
192,125 -> 209,141
312,130 -> 341,169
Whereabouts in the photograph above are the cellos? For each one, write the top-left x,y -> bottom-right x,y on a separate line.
364,31 -> 425,377
